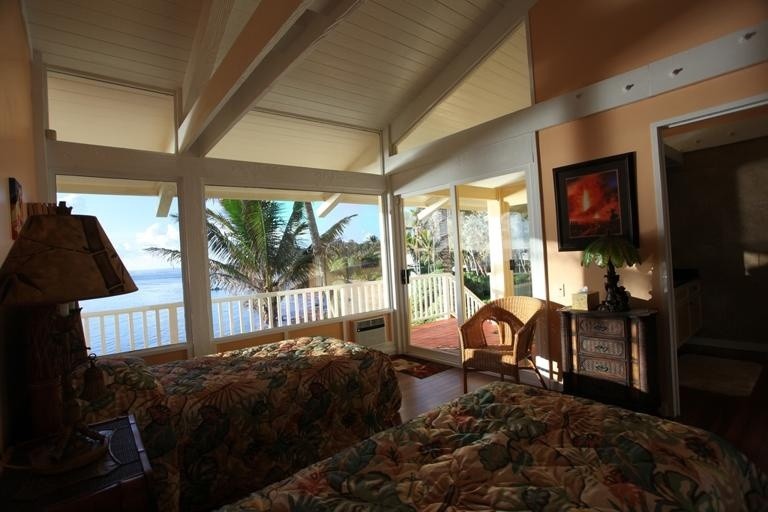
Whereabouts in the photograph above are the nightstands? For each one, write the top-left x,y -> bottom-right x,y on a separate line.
0,413 -> 152,512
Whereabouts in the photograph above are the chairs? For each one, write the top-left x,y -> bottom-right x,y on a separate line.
457,295 -> 550,395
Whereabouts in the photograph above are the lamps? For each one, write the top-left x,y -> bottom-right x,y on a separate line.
580,236 -> 643,314
0,216 -> 138,474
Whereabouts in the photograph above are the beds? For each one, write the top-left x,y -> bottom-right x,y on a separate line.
56,333 -> 404,512
211,382 -> 767,511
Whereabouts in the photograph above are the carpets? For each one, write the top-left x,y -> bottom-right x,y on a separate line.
390,352 -> 452,380
678,353 -> 764,397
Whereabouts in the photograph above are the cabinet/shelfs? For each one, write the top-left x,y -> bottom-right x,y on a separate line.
556,304 -> 665,416
676,285 -> 706,350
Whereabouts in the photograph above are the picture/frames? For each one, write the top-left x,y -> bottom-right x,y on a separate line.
8,177 -> 24,240
552,151 -> 639,251
